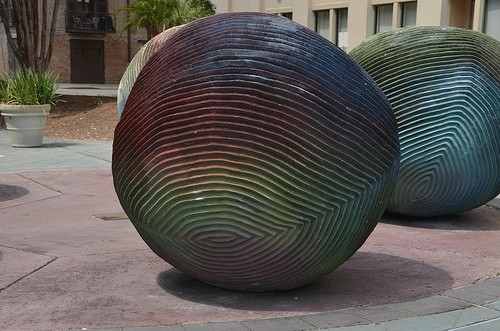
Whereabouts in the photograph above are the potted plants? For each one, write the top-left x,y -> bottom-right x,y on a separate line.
0,65 -> 67,148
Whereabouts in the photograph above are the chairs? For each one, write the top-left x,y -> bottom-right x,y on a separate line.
71,14 -> 84,28
